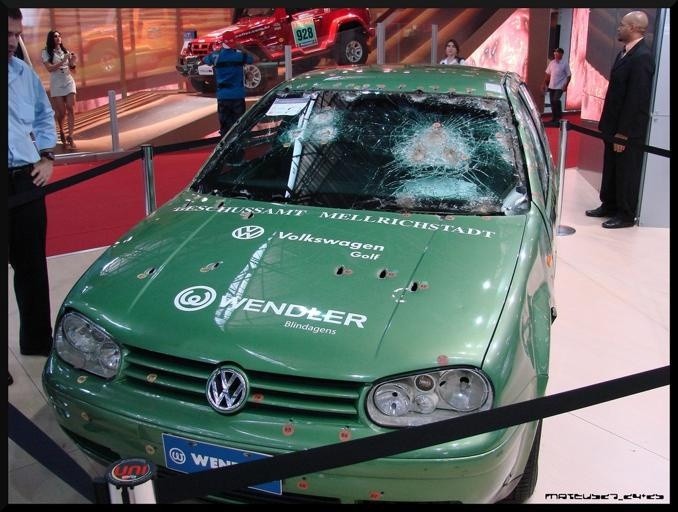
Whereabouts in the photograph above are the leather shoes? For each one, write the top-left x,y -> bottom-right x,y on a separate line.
20,341 -> 52,356
586,204 -> 616,217
602,216 -> 634,228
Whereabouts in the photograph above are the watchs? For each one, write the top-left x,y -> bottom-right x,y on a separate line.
41,152 -> 55,161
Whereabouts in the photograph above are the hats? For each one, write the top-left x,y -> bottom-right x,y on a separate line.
222,31 -> 237,49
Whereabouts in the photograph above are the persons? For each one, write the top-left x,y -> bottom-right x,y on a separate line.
440,40 -> 466,65
586,11 -> 656,229
42,31 -> 77,149
202,31 -> 260,167
545,48 -> 571,127
7,8 -> 57,385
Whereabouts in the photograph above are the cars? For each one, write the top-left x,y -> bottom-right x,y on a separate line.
175,3 -> 377,97
39,57 -> 561,508
